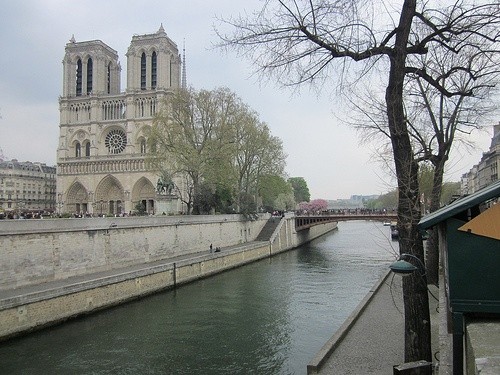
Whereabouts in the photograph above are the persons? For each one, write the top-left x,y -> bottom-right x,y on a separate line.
272,207 -> 398,217
0,209 -> 186,220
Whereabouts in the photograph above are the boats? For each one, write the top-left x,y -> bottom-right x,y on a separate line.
390,221 -> 399,241
383,221 -> 390,226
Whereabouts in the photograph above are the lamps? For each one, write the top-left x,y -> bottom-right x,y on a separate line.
390,253 -> 440,315
